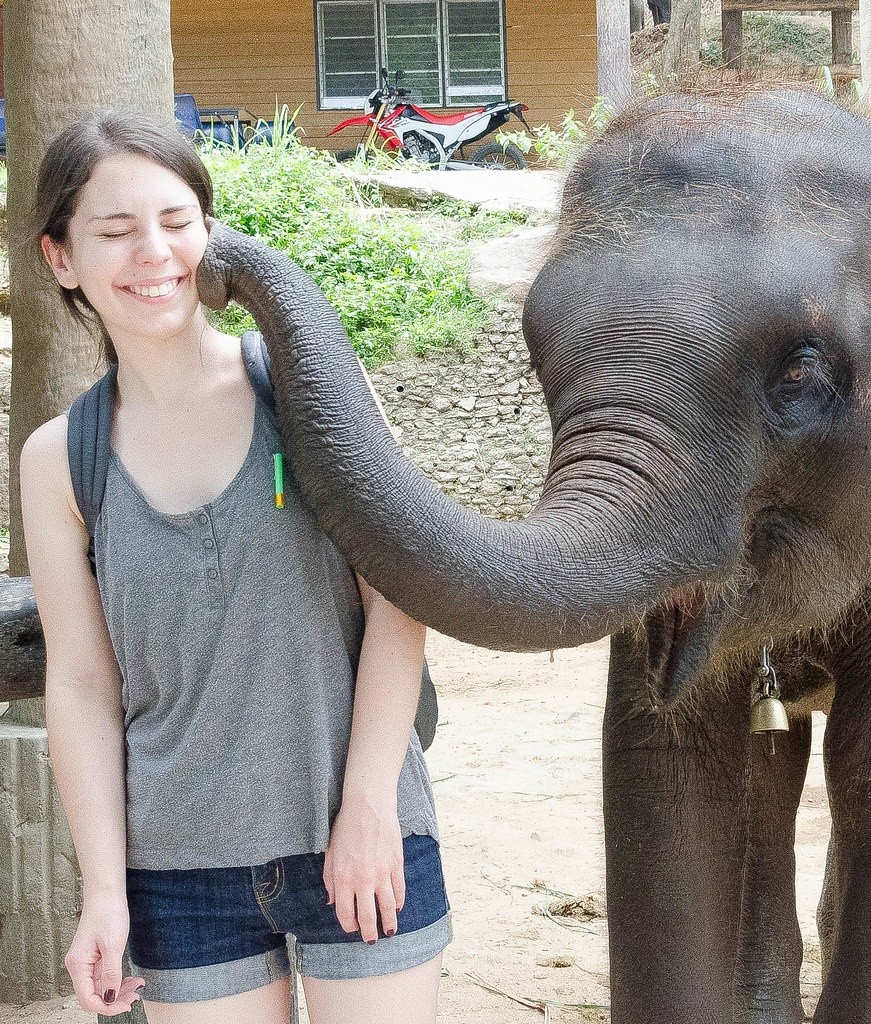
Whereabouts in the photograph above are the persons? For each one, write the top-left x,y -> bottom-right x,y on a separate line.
20,112 -> 452,1024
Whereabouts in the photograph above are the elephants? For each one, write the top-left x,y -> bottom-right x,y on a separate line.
194,88 -> 870,1023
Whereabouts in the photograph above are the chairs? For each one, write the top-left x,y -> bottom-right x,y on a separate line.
174,95 -> 299,149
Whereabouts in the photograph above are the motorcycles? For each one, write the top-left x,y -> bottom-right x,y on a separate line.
325,67 -> 537,171
